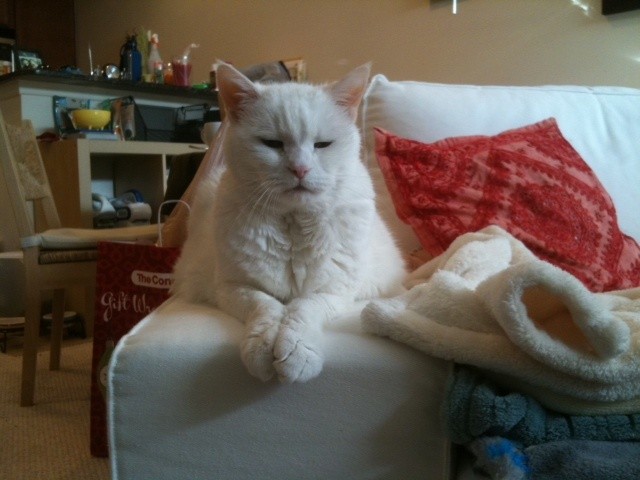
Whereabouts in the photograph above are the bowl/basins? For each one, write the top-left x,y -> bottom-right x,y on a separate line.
73,109 -> 111,131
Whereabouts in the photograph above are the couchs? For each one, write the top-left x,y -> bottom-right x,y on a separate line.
103,78 -> 640,480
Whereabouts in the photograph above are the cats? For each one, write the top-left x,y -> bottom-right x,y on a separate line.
167,61 -> 409,385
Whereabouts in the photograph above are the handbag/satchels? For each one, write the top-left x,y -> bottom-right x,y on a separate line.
88,197 -> 194,459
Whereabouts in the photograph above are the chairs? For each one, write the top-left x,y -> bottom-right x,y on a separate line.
2,120 -> 165,406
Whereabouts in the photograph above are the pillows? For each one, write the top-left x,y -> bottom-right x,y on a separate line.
373,119 -> 638,289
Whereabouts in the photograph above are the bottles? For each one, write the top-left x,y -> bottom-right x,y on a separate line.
145,33 -> 164,83
123,37 -> 142,82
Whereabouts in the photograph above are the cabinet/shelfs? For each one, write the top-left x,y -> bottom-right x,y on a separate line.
2,71 -> 218,340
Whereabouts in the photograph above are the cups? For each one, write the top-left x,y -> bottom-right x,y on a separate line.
171,55 -> 192,87
